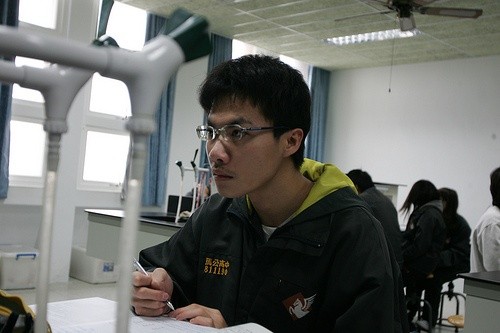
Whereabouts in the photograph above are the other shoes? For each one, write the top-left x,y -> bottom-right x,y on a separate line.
417,319 -> 435,333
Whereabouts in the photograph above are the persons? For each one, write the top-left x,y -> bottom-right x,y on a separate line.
345,168 -> 403,271
129,53 -> 395,333
470,167 -> 500,274
406,187 -> 471,326
398,180 -> 447,281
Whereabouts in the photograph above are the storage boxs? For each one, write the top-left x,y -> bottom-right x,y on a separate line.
69,244 -> 118,283
0,242 -> 39,290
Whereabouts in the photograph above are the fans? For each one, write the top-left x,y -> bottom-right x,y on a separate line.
333,0 -> 482,91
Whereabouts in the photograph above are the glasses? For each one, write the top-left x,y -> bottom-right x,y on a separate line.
196,123 -> 273,142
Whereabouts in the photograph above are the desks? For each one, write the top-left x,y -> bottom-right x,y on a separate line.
457,269 -> 500,332
85,209 -> 186,263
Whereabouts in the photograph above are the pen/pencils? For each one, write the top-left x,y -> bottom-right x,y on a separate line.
131,257 -> 175,311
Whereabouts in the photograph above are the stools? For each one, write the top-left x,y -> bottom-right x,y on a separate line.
404,275 -> 433,333
435,275 -> 466,333
